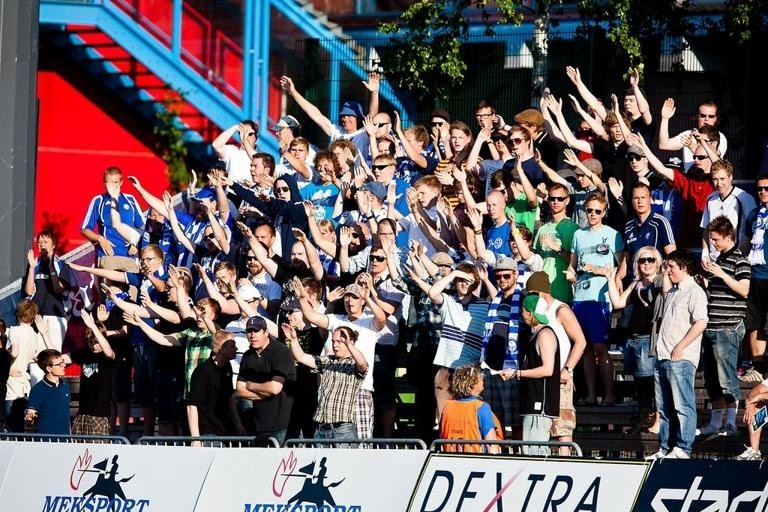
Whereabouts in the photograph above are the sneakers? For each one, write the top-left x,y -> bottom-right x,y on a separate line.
645,422 -> 762,461
737,369 -> 765,383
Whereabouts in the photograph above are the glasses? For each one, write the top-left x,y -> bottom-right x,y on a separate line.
204,232 -> 215,239
587,207 -> 603,215
627,112 -> 717,161
371,164 -> 388,171
758,185 -> 767,193
369,254 -> 385,263
637,256 -> 656,265
247,254 -> 257,262
274,186 -> 290,192
430,113 -> 521,144
52,359 -> 65,367
548,197 -> 568,204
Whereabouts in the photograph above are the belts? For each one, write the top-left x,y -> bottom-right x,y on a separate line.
318,422 -> 341,431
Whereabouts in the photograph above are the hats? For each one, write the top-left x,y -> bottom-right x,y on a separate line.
280,300 -> 301,310
191,190 -> 215,201
515,109 -> 544,126
495,257 -> 550,324
237,285 -> 262,301
344,284 -> 362,298
431,251 -> 453,266
363,182 -> 387,200
574,159 -> 602,177
276,115 -> 301,128
339,101 -> 364,118
245,316 -> 267,331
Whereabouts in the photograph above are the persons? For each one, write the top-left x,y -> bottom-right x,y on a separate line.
3,65 -> 768,464
